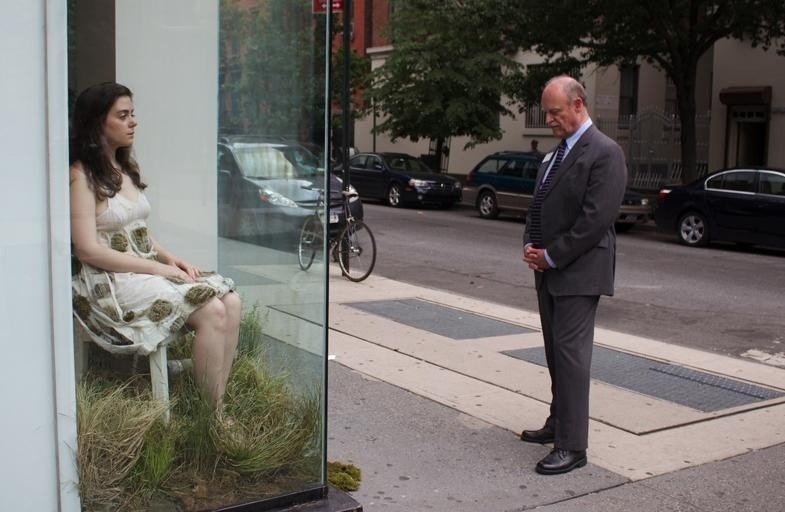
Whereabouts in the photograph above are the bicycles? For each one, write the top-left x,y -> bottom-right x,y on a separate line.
296,183 -> 377,281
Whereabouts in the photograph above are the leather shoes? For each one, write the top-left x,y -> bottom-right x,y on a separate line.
536,448 -> 587,475
521,416 -> 554,443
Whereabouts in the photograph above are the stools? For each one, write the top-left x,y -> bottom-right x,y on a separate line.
73,316 -> 192,429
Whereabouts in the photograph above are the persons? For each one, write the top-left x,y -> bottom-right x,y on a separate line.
519,75 -> 627,476
68,82 -> 242,427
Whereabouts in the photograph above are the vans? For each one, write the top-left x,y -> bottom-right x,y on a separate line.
216,136 -> 365,242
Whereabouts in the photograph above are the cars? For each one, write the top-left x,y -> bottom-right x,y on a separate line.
459,149 -> 653,237
334,150 -> 462,206
654,162 -> 784,252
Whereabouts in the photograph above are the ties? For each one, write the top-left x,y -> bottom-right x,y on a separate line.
529,139 -> 567,246
